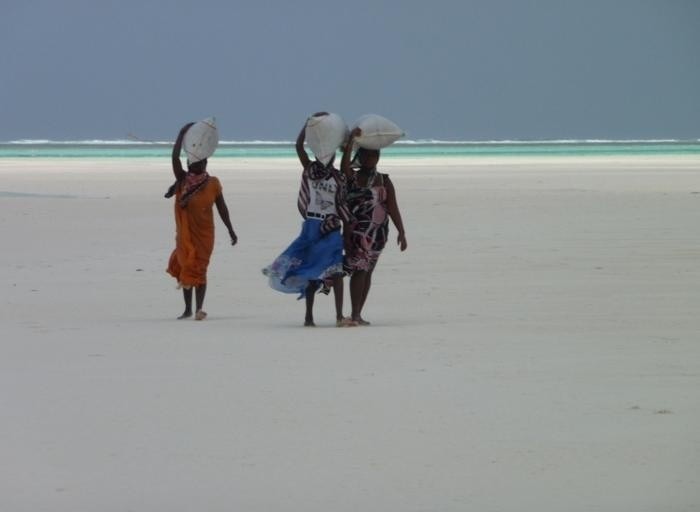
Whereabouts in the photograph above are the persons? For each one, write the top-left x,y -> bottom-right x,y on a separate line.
341,126 -> 407,325
264,112 -> 358,326
165,121 -> 238,322
